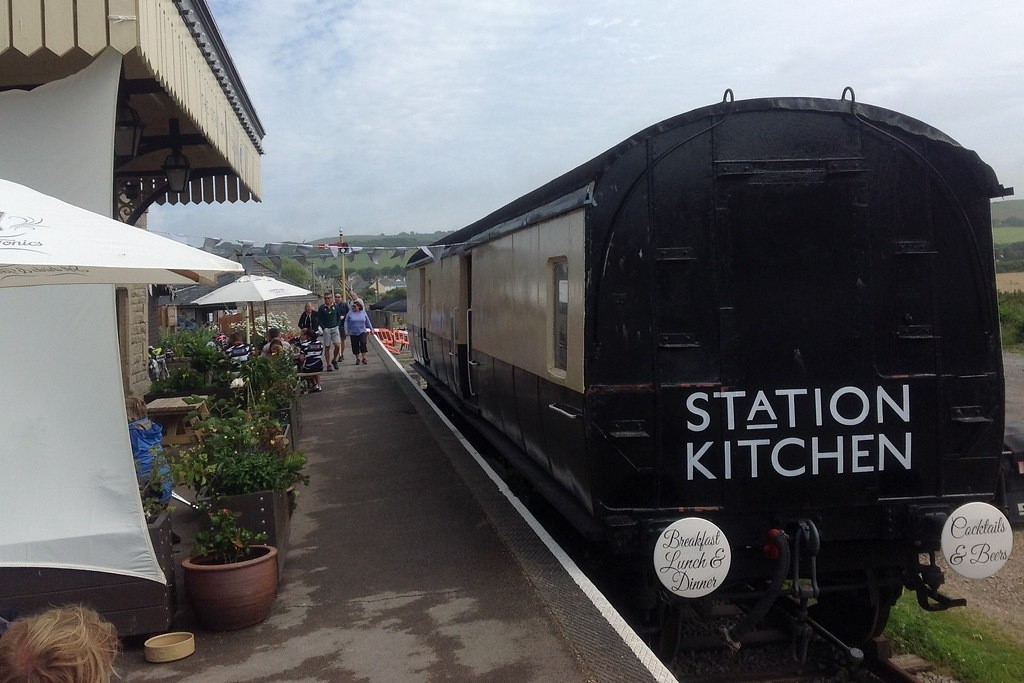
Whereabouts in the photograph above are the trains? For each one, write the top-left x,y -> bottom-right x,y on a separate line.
402,87 -> 1024,662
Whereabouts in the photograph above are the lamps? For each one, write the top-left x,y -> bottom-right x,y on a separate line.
159,148 -> 192,192
112,99 -> 148,160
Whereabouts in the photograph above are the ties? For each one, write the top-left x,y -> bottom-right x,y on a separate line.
306,315 -> 311,328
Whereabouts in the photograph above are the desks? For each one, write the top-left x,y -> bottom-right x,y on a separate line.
145,394 -> 213,446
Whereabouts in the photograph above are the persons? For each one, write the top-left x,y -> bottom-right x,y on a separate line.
0,602 -> 121,683
298,328 -> 324,391
224,326 -> 251,365
260,328 -> 295,357
298,290 -> 374,372
125,395 -> 172,504
262,339 -> 290,360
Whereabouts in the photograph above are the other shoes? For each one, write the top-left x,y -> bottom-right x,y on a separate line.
332,359 -> 339,369
356,359 -> 360,365
310,383 -> 318,392
327,364 -> 332,372
318,385 -> 322,390
339,356 -> 342,361
362,358 -> 367,364
341,354 -> 344,360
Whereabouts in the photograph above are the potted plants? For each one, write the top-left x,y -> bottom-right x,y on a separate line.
179,506 -> 279,632
150,322 -> 306,584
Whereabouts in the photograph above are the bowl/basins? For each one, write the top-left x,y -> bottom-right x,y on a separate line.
143,632 -> 195,663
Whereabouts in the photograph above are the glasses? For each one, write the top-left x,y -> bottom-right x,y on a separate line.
352,305 -> 356,307
325,297 -> 331,299
335,297 -> 340,298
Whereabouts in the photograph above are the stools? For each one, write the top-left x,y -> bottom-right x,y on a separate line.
296,372 -> 322,394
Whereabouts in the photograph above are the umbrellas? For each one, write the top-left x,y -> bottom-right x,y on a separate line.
1,178 -> 244,291
190,275 -> 313,357
